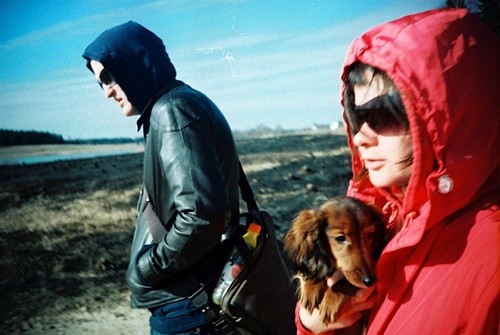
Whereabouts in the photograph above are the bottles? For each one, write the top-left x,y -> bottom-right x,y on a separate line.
212,223 -> 261,304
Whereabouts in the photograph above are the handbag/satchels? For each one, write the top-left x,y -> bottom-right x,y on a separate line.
220,209 -> 296,335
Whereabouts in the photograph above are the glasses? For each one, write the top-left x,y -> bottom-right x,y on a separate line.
350,91 -> 411,136
98,67 -> 114,90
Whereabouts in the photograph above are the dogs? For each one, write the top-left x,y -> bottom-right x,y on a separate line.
284,195 -> 381,326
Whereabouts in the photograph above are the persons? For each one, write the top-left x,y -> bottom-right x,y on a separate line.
294,8 -> 500,335
81,20 -> 240,335
